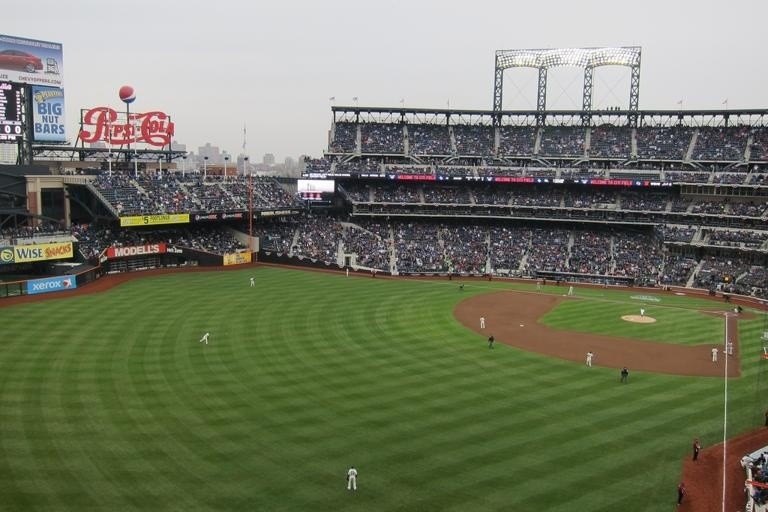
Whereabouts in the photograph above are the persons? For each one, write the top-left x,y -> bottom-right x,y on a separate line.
619,367 -> 628,383
691,437 -> 701,459
733,306 -> 738,314
1,124 -> 768,302
639,308 -> 645,321
764,410 -> 768,427
676,479 -> 686,504
344,465 -> 358,491
479,316 -> 487,328
488,334 -> 493,349
738,305 -> 742,313
710,346 -> 720,369
199,331 -> 209,344
748,451 -> 768,511
586,350 -> 593,367
726,340 -> 733,355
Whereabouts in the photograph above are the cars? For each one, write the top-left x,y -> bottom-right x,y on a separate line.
0,50 -> 43,74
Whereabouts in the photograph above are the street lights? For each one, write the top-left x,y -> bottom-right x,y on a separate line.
204,157 -> 208,178
225,156 -> 229,176
182,156 -> 187,178
133,154 -> 139,179
244,157 -> 248,176
109,153 -> 114,179
158,156 -> 164,176
